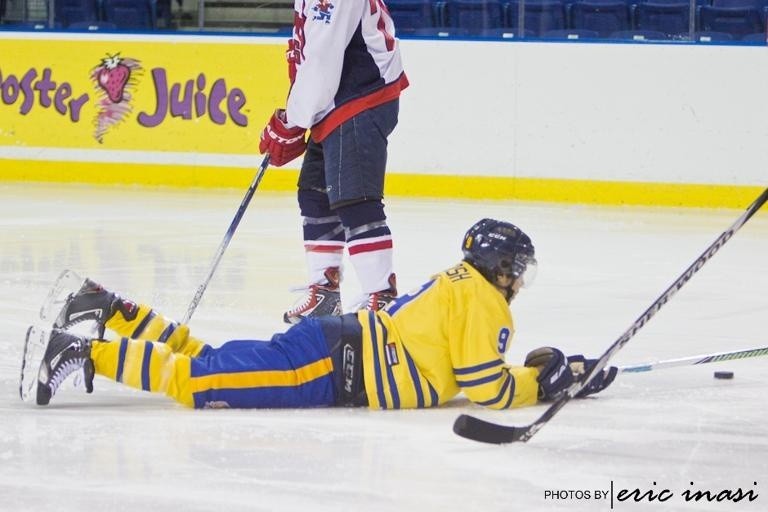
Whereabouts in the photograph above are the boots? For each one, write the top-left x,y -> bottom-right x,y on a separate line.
53,277 -> 140,340
283,266 -> 398,325
36,329 -> 95,405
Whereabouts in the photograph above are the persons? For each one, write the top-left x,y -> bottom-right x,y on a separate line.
36,217 -> 619,411
258,0 -> 410,325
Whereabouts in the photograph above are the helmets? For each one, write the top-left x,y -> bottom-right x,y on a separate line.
462,219 -> 537,279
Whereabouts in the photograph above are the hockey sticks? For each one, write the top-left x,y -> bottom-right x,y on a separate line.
453,190 -> 767,442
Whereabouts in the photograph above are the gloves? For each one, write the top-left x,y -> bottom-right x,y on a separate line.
259,108 -> 308,167
524,346 -> 618,402
